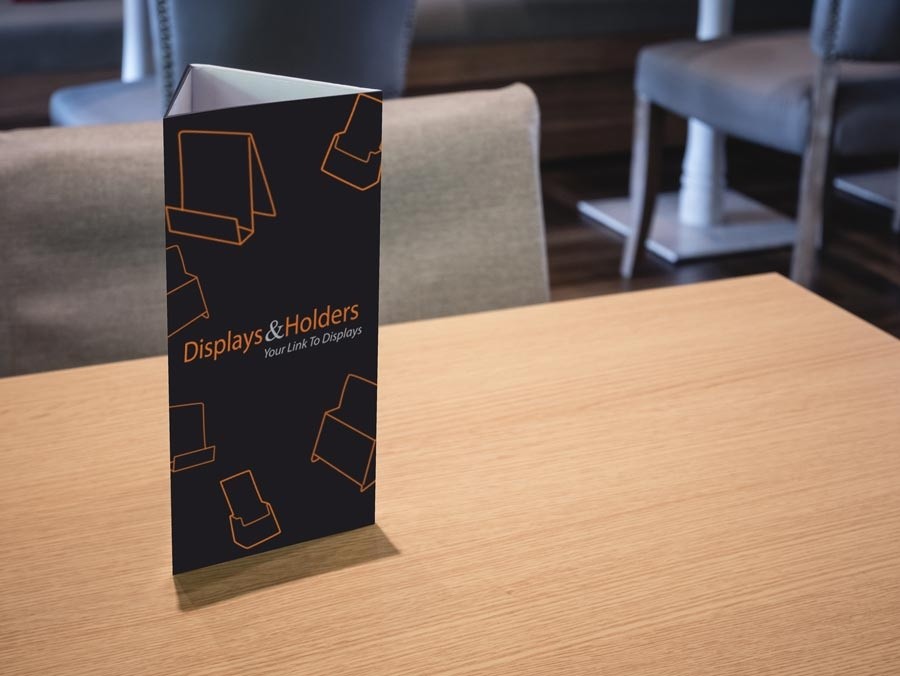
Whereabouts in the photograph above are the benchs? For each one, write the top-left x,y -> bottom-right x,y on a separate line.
0,80 -> 553,379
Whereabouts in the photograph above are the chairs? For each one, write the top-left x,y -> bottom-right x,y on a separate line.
48,0 -> 420,130
619,0 -> 900,297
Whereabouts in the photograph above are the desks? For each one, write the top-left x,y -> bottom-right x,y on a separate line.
0,272 -> 900,676
